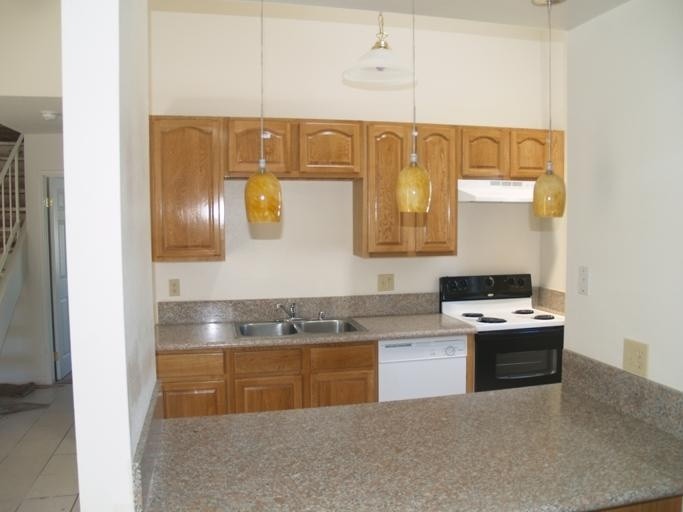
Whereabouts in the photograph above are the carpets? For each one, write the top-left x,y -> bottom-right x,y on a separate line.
0,400 -> 51,416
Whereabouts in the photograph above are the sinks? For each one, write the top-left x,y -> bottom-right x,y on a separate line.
291,317 -> 369,334
232,318 -> 298,336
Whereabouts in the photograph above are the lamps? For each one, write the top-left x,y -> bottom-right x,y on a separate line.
343,11 -> 419,84
393,2 -> 434,213
243,2 -> 283,223
531,0 -> 568,219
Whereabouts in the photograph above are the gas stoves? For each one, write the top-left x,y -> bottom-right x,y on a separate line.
441,297 -> 566,327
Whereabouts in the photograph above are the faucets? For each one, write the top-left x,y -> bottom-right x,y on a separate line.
275,301 -> 302,319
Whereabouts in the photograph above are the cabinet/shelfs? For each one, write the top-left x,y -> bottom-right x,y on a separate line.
225,118 -> 363,178
305,344 -> 375,410
153,350 -> 227,418
228,348 -> 305,417
149,115 -> 225,261
455,126 -> 564,182
352,122 -> 457,257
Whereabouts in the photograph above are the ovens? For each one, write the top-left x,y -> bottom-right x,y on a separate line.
474,326 -> 563,393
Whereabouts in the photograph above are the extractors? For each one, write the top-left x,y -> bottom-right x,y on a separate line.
457,179 -> 537,205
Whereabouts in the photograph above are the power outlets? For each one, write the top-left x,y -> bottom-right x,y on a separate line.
621,337 -> 648,377
